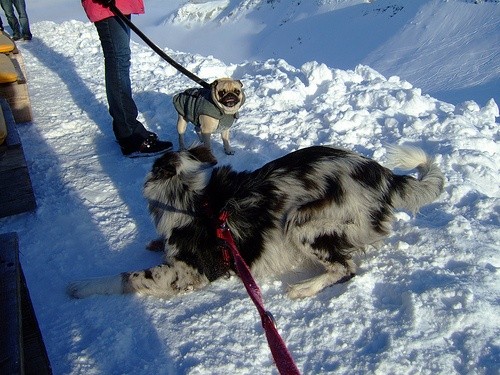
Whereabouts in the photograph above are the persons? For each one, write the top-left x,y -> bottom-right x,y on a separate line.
81,0 -> 174,157
0,0 -> 32,43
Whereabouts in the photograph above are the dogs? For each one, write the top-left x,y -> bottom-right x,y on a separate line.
172,78 -> 246,155
67,140 -> 444,300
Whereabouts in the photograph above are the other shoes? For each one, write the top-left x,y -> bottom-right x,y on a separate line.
22,34 -> 32,40
12,32 -> 22,40
120,131 -> 174,158
